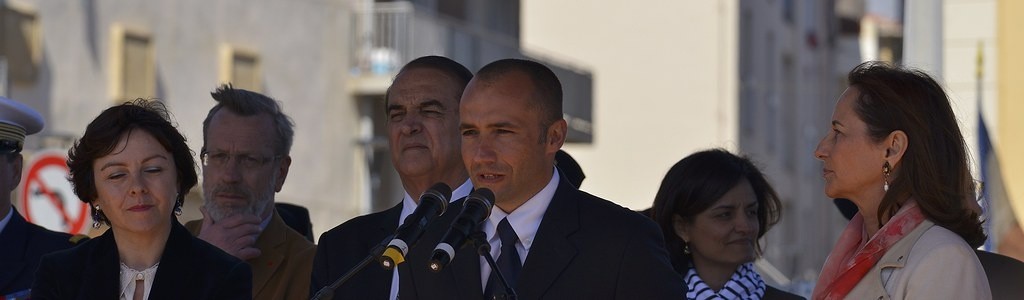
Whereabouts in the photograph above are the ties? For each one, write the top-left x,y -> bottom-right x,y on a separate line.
484,218 -> 522,300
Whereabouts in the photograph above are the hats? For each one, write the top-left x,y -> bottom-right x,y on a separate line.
0,94 -> 44,156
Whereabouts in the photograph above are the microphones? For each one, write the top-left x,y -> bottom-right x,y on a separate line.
380,182 -> 452,270
428,187 -> 495,273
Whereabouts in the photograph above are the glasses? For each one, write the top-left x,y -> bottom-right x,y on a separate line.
200,149 -> 285,171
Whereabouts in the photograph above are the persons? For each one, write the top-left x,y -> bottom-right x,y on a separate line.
0,56 -> 807,300
811,61 -> 1024,300
